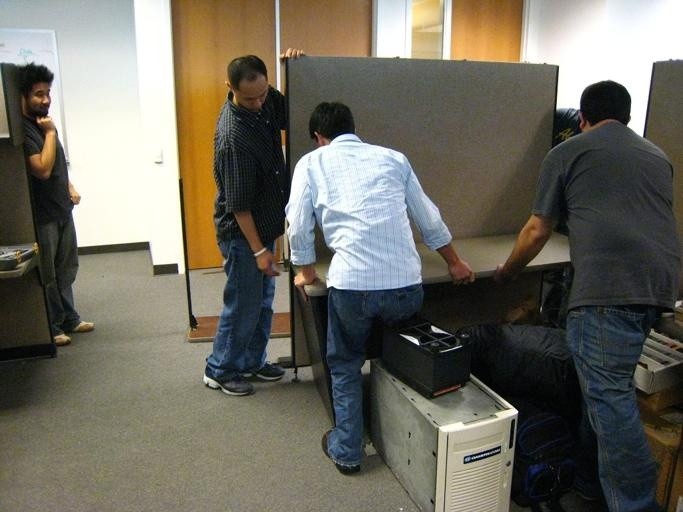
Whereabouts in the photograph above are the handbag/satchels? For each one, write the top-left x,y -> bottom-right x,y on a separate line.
511,411 -> 577,512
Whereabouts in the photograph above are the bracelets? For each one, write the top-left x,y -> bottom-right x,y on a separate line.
254,246 -> 267,258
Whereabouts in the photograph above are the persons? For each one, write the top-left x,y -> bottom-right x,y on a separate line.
18,62 -> 96,346
202,46 -> 306,397
496,77 -> 683,511
282,101 -> 475,474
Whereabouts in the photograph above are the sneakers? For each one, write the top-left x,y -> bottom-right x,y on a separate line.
322,430 -> 360,475
63,321 -> 96,333
52,333 -> 72,346
236,361 -> 285,381
203,357 -> 256,396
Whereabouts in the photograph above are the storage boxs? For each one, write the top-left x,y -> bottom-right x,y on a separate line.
639,302 -> 682,512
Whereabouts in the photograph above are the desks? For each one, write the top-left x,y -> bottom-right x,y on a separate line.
0,59 -> 62,365
279,52 -> 571,425
